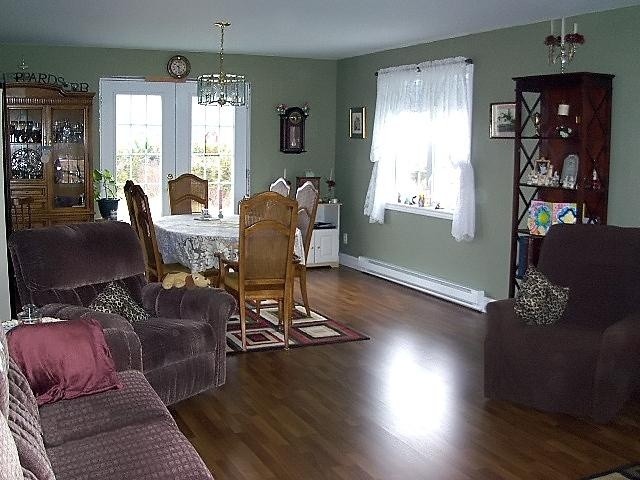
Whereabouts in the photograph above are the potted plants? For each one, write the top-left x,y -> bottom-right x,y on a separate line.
92,170 -> 120,218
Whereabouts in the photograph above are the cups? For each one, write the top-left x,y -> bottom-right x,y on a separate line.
331,198 -> 338,203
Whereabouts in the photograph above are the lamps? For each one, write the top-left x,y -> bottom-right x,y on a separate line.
197,22 -> 245,106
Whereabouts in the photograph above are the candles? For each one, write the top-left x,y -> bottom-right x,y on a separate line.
284,167 -> 286,178
550,16 -> 577,42
329,167 -> 333,180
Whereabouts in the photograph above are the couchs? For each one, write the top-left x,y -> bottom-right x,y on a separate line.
0,314 -> 215,479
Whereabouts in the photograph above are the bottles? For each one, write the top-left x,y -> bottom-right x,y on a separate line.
79,195 -> 84,205
10,119 -> 84,143
54,159 -> 62,184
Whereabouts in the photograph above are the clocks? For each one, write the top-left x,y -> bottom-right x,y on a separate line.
167,55 -> 191,78
275,104 -> 308,154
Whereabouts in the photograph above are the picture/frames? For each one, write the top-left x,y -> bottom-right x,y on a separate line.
490,102 -> 514,139
350,107 -> 365,139
534,156 -> 550,175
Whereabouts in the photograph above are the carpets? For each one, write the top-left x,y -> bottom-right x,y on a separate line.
145,263 -> 371,352
582,462 -> 640,480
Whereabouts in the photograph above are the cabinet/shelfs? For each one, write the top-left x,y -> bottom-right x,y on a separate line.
509,72 -> 614,304
2,61 -> 95,229
306,229 -> 339,268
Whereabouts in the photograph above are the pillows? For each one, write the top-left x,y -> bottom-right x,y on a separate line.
514,263 -> 570,329
89,281 -> 150,324
8,318 -> 121,403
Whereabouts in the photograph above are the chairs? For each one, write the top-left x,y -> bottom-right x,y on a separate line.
10,197 -> 34,229
124,174 -> 319,352
8,220 -> 237,407
482,224 -> 640,425
